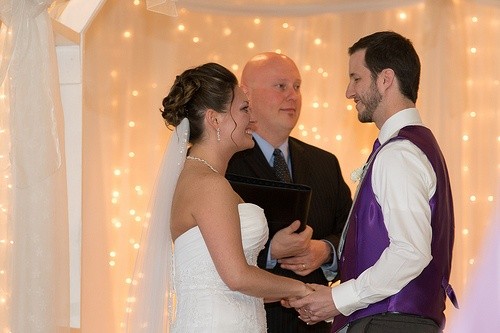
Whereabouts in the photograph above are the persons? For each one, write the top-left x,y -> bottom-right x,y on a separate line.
224,52 -> 353,333
131,62 -> 320,333
280,31 -> 459,333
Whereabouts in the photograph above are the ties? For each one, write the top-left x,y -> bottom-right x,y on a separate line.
362,138 -> 382,171
272,148 -> 292,184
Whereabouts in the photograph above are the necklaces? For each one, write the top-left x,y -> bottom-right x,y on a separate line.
186,155 -> 218,173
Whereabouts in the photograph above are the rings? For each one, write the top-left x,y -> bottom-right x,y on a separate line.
302,264 -> 306,270
